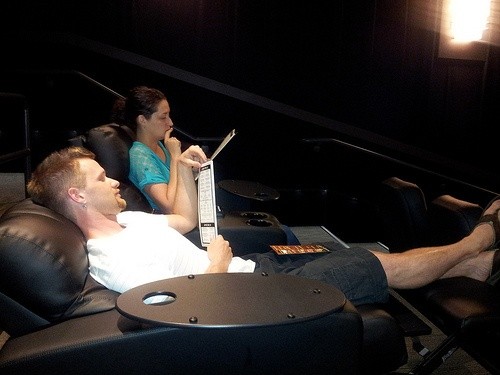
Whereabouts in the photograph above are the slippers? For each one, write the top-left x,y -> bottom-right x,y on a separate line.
468,195 -> 500,250
485,250 -> 500,288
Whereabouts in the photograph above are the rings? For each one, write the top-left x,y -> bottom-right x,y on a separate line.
171,137 -> 174,138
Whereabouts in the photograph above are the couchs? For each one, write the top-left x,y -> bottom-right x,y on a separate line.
376,176 -> 500,375
70,122 -> 288,258
1,198 -> 408,375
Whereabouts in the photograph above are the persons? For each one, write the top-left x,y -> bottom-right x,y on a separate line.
114,86 -> 318,227
25,146 -> 500,308
